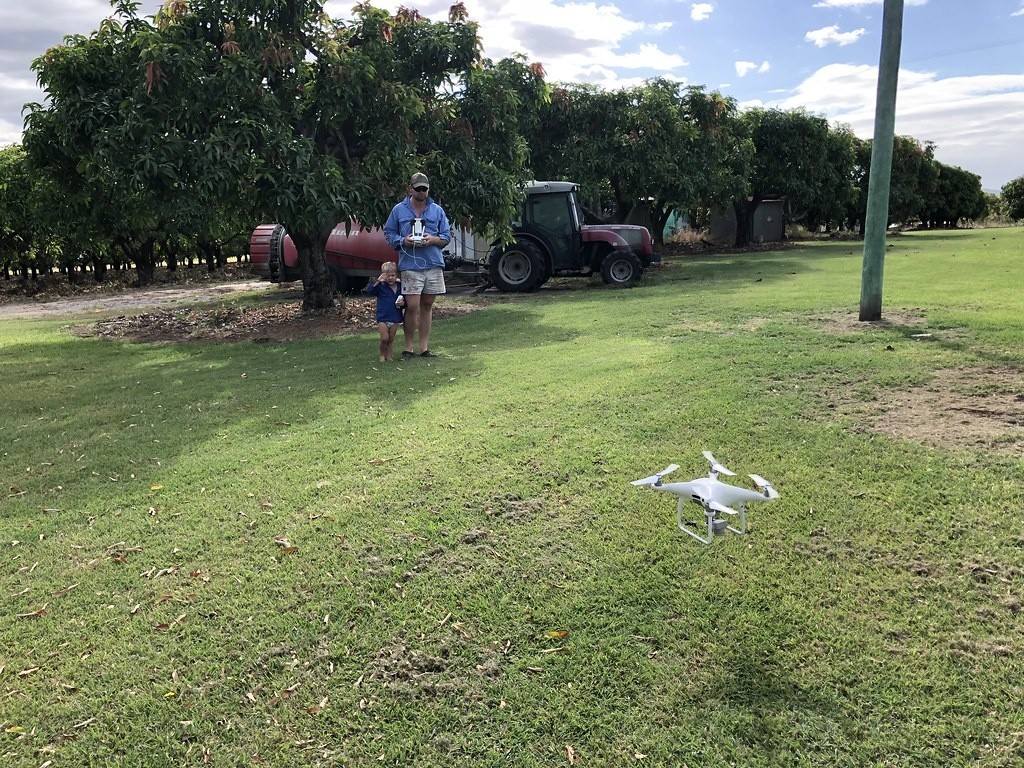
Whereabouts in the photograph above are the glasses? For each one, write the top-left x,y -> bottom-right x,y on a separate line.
412,185 -> 428,192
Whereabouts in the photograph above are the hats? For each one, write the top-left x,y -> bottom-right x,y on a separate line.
410,172 -> 429,189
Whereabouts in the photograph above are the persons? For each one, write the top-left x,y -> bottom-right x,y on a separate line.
366,262 -> 405,363
384,172 -> 451,363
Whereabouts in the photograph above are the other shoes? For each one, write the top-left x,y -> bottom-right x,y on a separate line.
399,350 -> 413,361
420,350 -> 438,357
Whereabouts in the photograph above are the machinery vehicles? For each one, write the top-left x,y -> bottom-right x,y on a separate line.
249,179 -> 660,295
